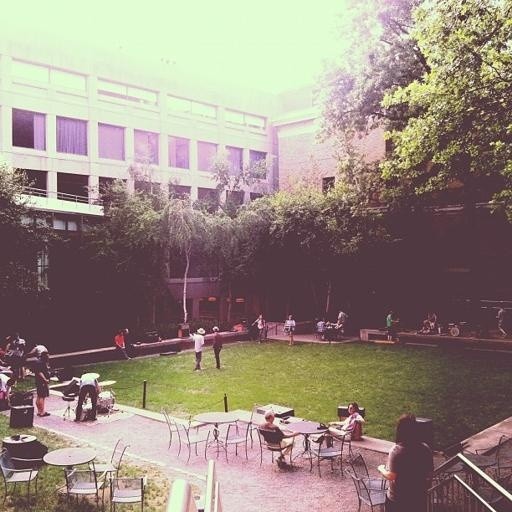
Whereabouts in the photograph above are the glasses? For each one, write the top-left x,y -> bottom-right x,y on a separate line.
320,423 -> 326,427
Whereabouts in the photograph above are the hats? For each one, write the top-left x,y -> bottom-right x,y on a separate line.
196,328 -> 206,334
213,327 -> 219,332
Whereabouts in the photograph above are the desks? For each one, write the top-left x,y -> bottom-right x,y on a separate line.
2,434 -> 49,468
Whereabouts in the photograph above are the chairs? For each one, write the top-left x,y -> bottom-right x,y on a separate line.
0,447 -> 42,507
345,435 -> 511,512
161,407 -> 358,479
64,460 -> 108,511
90,439 -> 131,485
110,478 -> 148,511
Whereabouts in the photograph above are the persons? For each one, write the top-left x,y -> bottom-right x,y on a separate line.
496,305 -> 508,339
252,314 -> 266,344
417,312 -> 438,335
189,328 -> 205,370
377,413 -> 433,512
314,310 -> 349,341
258,411 -> 300,466
386,310 -> 400,342
0,333 -> 50,417
74,373 -> 101,421
312,402 -> 366,448
282,314 -> 295,346
213,327 -> 222,369
114,329 -> 131,359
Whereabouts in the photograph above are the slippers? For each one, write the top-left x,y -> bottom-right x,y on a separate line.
37,412 -> 50,417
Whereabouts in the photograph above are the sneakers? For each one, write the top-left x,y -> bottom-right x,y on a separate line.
312,439 -> 322,444
276,457 -> 286,466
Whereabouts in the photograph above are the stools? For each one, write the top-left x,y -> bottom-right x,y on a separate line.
61,395 -> 77,421
